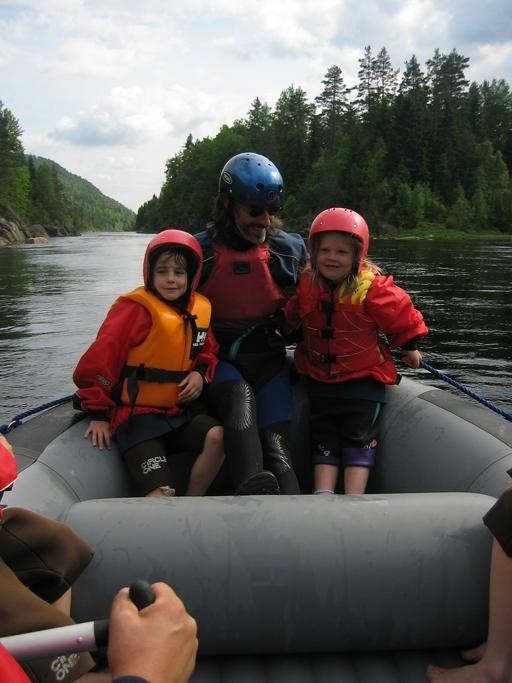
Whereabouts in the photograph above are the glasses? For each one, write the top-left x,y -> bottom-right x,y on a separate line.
233,200 -> 280,217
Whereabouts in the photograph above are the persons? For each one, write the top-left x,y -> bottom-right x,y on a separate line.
1,425 -> 199,682
425,470 -> 512,683
187,152 -> 313,496
281,207 -> 429,494
68,229 -> 225,497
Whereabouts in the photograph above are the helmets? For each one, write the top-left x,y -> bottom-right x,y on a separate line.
308,206 -> 369,272
217,151 -> 284,203
142,228 -> 205,294
